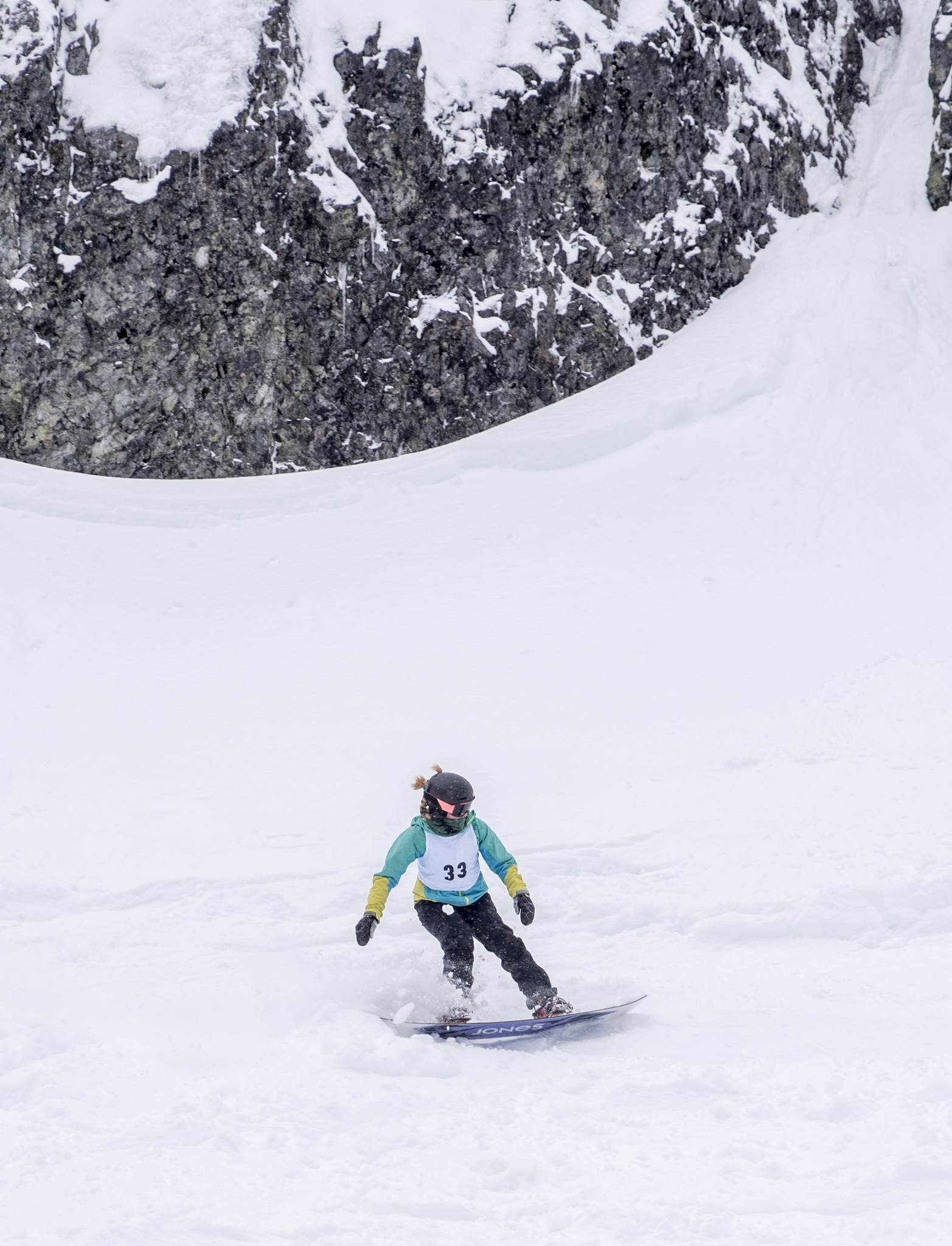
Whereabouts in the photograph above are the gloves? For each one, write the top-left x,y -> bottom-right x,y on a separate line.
356,911 -> 377,946
514,893 -> 535,926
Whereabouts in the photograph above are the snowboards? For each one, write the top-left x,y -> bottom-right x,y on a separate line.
379,992 -> 649,1040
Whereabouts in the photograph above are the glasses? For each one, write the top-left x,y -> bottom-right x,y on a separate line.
452,801 -> 472,817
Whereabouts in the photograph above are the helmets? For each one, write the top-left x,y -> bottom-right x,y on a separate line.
424,772 -> 475,833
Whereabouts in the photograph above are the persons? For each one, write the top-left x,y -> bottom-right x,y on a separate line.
355,764 -> 573,1027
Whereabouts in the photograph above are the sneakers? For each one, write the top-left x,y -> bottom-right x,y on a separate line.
435,1004 -> 471,1024
532,995 -> 573,1019
